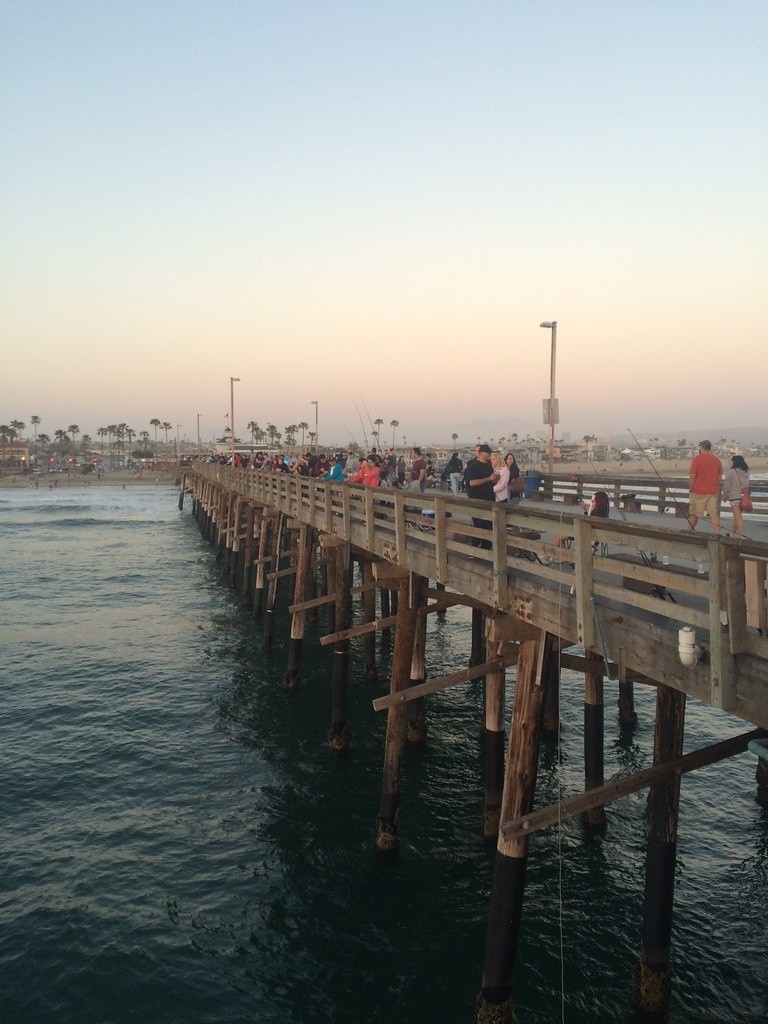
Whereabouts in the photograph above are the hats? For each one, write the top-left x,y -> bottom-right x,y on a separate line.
329,456 -> 336,462
479,444 -> 495,453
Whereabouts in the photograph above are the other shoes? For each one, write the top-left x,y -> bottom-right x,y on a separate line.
725,532 -> 731,537
534,555 -> 553,565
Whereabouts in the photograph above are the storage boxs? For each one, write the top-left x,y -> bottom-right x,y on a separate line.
422,510 -> 452,531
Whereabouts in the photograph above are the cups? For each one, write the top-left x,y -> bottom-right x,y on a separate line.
650,552 -> 657,562
698,563 -> 704,573
663,556 -> 668,565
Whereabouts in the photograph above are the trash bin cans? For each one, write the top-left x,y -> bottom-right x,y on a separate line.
523,470 -> 541,498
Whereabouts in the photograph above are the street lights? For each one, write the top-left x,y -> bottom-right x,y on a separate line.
197,413 -> 203,458
311,401 -> 319,453
230,377 -> 240,466
539,321 -> 557,472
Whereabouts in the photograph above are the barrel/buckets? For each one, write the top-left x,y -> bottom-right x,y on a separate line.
422,510 -> 435,531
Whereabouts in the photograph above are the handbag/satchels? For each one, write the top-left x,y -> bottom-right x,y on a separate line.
510,475 -> 525,493
740,488 -> 753,513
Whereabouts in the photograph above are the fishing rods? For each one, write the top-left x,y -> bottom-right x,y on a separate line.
590,426 -> 753,604
340,395 -> 403,490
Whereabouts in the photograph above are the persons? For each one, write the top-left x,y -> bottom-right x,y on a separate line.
460,444 -> 527,560
687,440 -> 723,534
202,447 -> 463,524
722,455 -> 753,540
534,492 -> 610,566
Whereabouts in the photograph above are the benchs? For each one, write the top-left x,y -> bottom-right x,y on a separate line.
607,552 -> 710,597
581,494 -> 690,518
449,518 -> 541,556
530,489 -> 579,506
